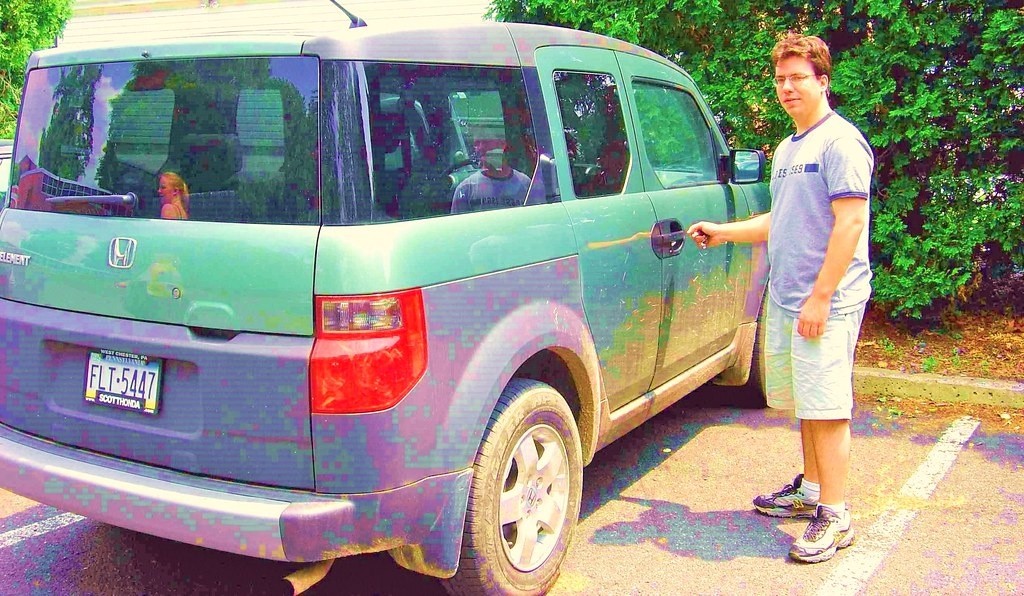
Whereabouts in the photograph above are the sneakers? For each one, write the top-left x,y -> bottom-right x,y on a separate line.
787,503 -> 855,562
752,473 -> 817,518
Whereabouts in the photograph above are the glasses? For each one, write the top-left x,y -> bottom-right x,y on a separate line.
772,74 -> 816,86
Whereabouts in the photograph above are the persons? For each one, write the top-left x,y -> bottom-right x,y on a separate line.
158,172 -> 192,219
688,37 -> 876,562
450,126 -> 535,214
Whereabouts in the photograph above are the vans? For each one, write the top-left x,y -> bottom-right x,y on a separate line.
0,21 -> 775,596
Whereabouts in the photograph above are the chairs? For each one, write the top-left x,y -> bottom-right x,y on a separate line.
157,94 -> 436,225
